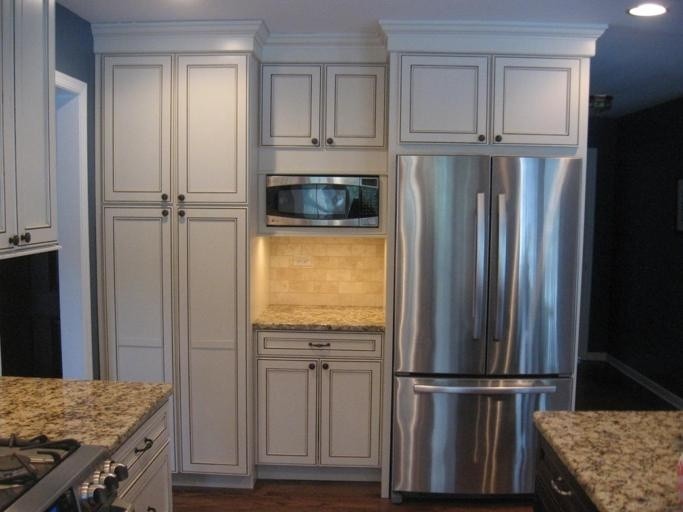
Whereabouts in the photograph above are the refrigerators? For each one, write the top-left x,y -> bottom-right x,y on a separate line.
391,155 -> 582,494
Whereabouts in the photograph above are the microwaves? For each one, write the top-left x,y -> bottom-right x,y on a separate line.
265,173 -> 380,228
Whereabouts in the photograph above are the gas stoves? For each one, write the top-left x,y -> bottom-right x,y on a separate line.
0,431 -> 130,512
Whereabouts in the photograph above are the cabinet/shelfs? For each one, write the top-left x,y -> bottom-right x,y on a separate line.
256,20 -> 387,156
1,1 -> 58,254
96,205 -> 258,490
253,327 -> 382,484
378,19 -> 605,158
93,17 -> 266,204
536,431 -> 597,512
109,406 -> 173,512
